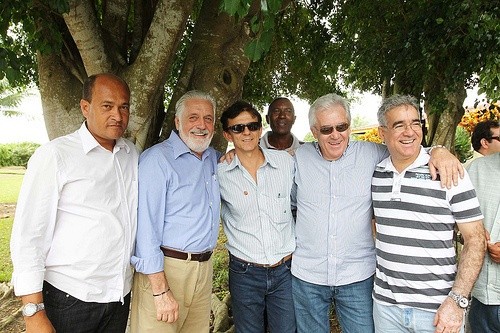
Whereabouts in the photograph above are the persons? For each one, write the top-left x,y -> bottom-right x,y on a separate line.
12,72 -> 139,333
128,90 -> 226,333
216,94 -> 500,333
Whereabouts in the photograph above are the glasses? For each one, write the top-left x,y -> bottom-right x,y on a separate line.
485,136 -> 500,142
381,121 -> 424,132
314,122 -> 350,135
227,122 -> 262,134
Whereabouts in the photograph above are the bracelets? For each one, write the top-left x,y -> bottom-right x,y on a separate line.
153,289 -> 170,296
429,145 -> 445,155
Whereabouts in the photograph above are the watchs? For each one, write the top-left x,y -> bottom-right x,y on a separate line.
447,291 -> 470,308
22,302 -> 45,317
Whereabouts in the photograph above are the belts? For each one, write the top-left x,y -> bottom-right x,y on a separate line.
236,254 -> 291,268
160,246 -> 213,262
